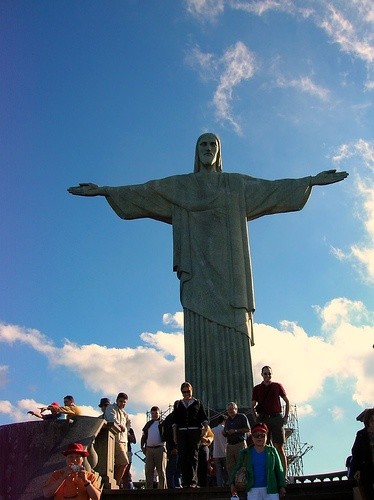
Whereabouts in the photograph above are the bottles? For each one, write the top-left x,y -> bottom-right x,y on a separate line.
231,493 -> 239,500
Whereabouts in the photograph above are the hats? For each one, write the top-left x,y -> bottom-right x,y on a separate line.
62,443 -> 88,457
48,402 -> 59,407
251,423 -> 268,433
98,398 -> 110,405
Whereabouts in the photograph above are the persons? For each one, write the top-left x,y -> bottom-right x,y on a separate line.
196,425 -> 214,487
103,393 -> 137,489
171,381 -> 209,488
211,415 -> 230,488
42,442 -> 104,500
27,395 -> 80,420
140,400 -> 183,489
348,408 -> 374,500
97,398 -> 110,419
221,402 -> 251,477
68,134 -> 348,407
228,423 -> 287,500
251,366 -> 291,483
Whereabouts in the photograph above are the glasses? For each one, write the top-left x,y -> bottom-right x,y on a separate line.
252,433 -> 265,438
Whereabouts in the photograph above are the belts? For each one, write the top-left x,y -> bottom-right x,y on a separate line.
147,445 -> 162,448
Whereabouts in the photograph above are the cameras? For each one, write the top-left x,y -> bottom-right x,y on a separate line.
70,465 -> 81,472
